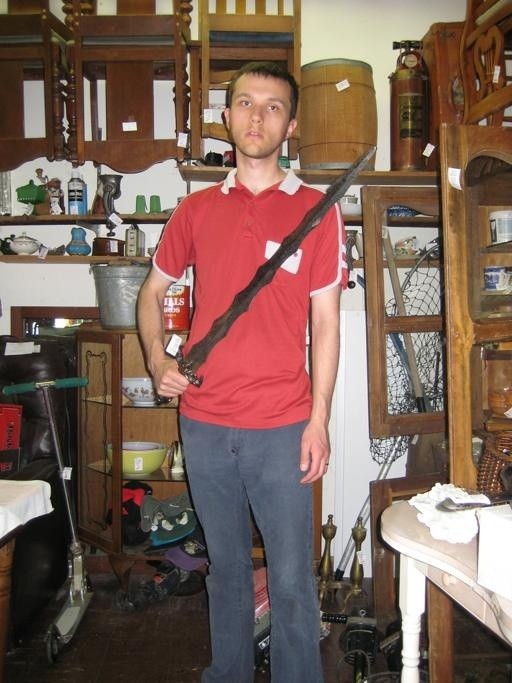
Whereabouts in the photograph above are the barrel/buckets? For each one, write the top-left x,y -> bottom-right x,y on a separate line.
300,58 -> 378,172
162,265 -> 190,331
87,264 -> 152,330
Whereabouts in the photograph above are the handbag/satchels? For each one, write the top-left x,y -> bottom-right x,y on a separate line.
106,482 -> 153,547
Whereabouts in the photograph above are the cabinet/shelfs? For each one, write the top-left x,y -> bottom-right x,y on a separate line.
0,213 -> 442,269
73,329 -> 191,556
359,22 -> 512,683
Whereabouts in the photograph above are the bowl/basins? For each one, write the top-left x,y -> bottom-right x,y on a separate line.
390,248 -> 422,259
122,378 -> 167,405
104,440 -> 167,480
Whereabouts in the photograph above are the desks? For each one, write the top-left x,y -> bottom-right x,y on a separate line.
0,480 -> 55,683
381,490 -> 512,683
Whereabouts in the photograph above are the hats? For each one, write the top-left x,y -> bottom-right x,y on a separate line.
164,531 -> 209,571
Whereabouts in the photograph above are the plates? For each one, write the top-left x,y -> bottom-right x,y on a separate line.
384,208 -> 423,219
339,203 -> 363,216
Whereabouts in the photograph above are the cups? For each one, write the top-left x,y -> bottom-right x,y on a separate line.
484,267 -> 511,291
489,211 -> 512,243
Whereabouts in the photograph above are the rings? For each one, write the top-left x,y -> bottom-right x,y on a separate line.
325,462 -> 329,468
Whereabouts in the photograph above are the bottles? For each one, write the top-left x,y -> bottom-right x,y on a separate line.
67,170 -> 87,214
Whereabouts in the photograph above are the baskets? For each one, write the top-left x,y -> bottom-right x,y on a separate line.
477,430 -> 512,499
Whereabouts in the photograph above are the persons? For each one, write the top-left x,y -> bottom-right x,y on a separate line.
137,64 -> 349,682
